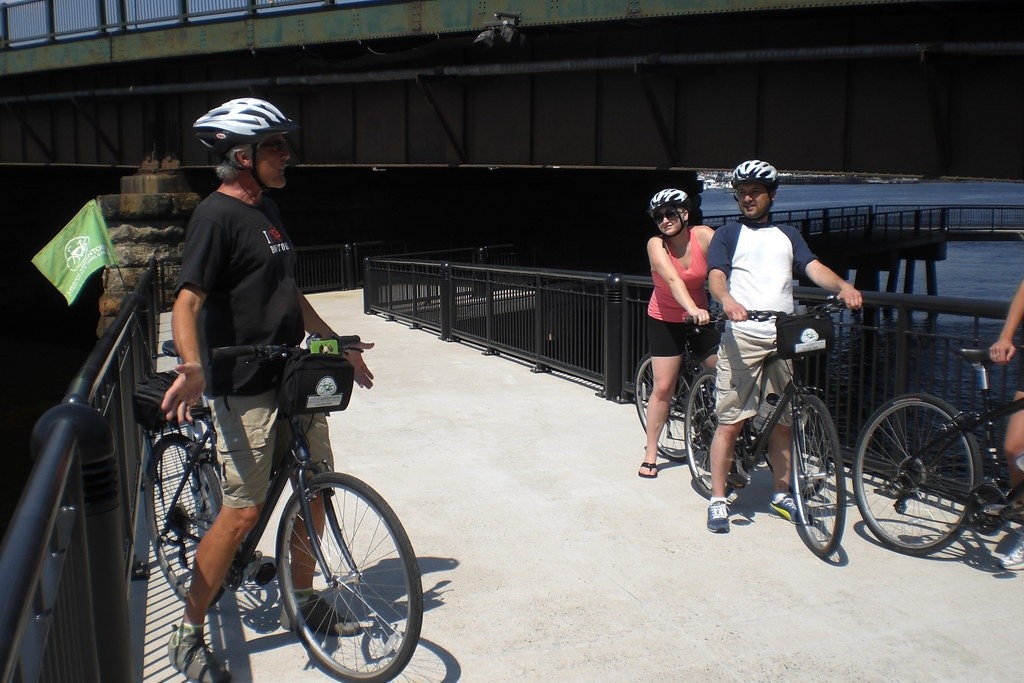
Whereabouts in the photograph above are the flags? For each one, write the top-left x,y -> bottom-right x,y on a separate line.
31,198 -> 120,307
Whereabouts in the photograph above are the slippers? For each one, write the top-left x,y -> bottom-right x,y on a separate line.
638,462 -> 658,478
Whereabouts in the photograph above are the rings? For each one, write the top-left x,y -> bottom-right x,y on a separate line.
994,354 -> 999,358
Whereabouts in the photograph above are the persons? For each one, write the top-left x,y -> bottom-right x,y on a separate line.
161,98 -> 375,683
990,277 -> 1024,570
707,160 -> 862,533
638,188 -> 719,478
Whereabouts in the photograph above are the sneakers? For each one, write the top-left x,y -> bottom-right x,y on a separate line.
280,592 -> 360,639
167,629 -> 232,683
999,540 -> 1024,571
768,494 -> 814,525
706,497 -> 731,534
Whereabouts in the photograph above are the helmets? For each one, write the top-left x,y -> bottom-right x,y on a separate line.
648,188 -> 688,211
730,160 -> 779,189
191,98 -> 300,150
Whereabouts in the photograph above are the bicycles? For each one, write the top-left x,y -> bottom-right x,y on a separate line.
852,346 -> 1024,555
626,292 -> 848,557
133,338 -> 423,683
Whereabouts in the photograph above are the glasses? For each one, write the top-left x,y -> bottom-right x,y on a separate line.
260,139 -> 287,151
654,211 -> 682,224
